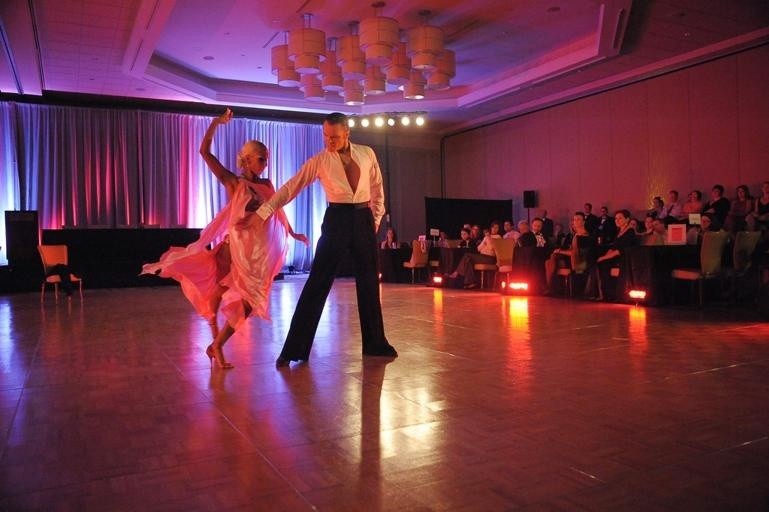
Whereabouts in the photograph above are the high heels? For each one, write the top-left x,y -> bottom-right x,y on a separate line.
207,346 -> 235,369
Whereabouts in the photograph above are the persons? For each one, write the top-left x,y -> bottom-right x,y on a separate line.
200,109 -> 311,369
436,210 -> 555,289
549,182 -> 769,301
381,229 -> 396,250
235,113 -> 397,367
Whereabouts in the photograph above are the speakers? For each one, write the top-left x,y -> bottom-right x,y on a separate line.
5,210 -> 38,266
523,190 -> 534,208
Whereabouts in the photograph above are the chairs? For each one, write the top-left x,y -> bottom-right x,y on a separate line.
40,304 -> 84,345
37,243 -> 85,304
377,229 -> 761,308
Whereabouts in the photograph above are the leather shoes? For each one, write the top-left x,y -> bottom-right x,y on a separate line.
390,347 -> 398,358
276,356 -> 292,366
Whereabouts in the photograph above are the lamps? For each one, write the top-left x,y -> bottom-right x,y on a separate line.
271,2 -> 456,129
